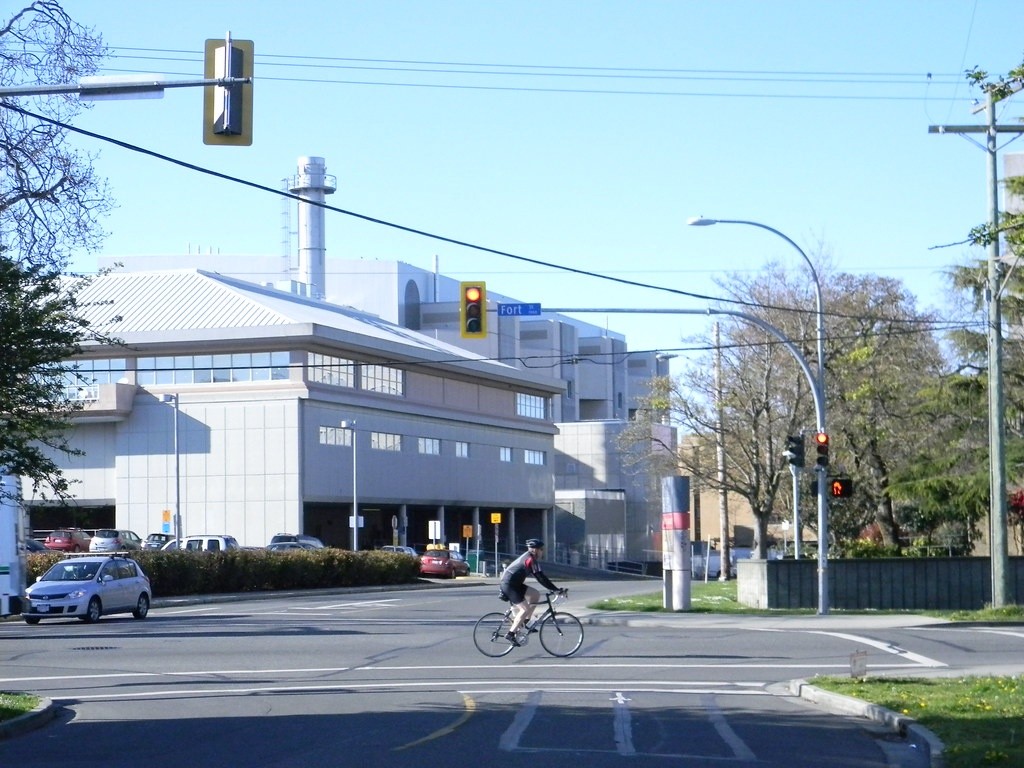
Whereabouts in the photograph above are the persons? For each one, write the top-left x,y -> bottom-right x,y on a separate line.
499,539 -> 564,647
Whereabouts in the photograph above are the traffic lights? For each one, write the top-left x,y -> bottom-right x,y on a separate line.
460,280 -> 487,339
816,432 -> 830,467
831,477 -> 853,497
787,435 -> 804,469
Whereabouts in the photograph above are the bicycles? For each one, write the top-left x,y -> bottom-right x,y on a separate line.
472,587 -> 584,657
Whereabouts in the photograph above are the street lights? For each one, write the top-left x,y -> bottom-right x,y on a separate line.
159,391 -> 179,549
689,218 -> 830,614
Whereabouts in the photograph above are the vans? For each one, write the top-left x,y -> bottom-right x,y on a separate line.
145,533 -> 176,552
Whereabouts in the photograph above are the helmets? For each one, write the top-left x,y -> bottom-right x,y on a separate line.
526,539 -> 544,548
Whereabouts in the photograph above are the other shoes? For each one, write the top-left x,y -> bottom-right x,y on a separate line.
505,634 -> 521,647
524,624 -> 538,632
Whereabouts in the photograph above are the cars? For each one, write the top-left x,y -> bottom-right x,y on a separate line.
160,534 -> 239,551
22,554 -> 152,625
419,548 -> 470,579
381,545 -> 418,561
265,534 -> 323,552
89,528 -> 145,555
43,529 -> 92,554
